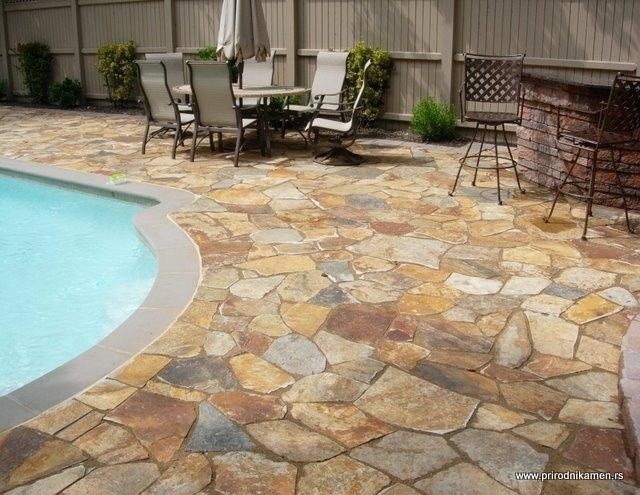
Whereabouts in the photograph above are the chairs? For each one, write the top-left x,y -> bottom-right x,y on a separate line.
447,52 -> 527,206
132,48 -> 373,168
542,68 -> 640,241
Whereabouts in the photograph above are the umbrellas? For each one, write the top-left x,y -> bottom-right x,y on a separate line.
216,0 -> 271,108
